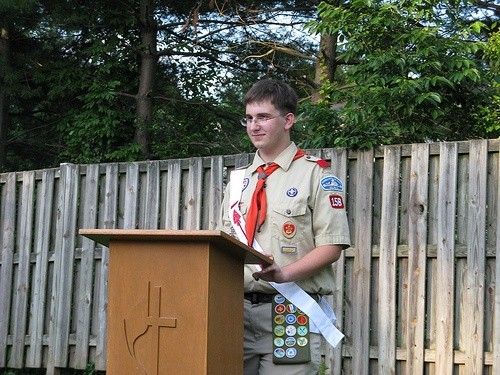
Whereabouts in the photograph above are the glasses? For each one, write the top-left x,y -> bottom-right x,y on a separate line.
240,113 -> 287,126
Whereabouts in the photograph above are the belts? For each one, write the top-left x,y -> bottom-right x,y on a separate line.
244,291 -> 272,303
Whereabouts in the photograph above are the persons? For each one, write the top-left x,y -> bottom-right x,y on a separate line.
214,79 -> 350,375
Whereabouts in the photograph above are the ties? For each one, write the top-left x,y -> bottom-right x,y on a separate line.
245,148 -> 304,247
